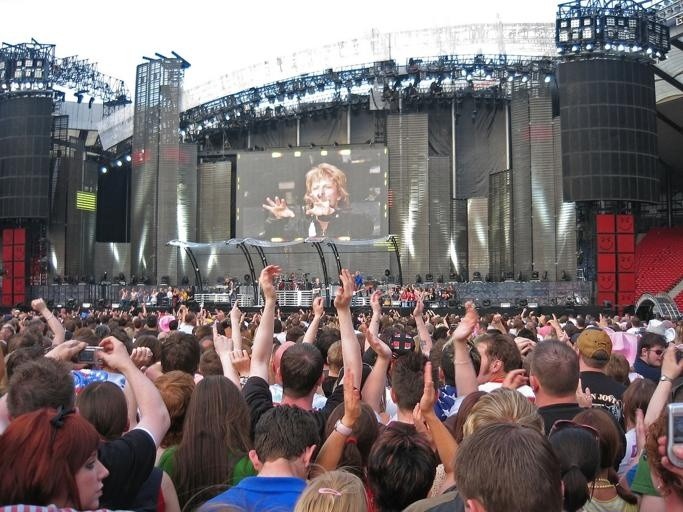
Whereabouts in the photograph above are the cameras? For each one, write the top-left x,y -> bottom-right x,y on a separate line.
74,345 -> 105,366
676,351 -> 683,358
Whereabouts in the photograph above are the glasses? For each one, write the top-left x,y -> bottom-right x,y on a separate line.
648,349 -> 664,354
548,417 -> 602,455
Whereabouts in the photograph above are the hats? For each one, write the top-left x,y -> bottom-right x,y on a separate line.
159,315 -> 178,332
577,329 -> 612,359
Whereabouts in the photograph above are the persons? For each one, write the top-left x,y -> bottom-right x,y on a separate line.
263,163 -> 375,242
0,264 -> 682,511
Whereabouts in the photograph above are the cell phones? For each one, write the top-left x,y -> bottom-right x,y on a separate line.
666,403 -> 683,471
